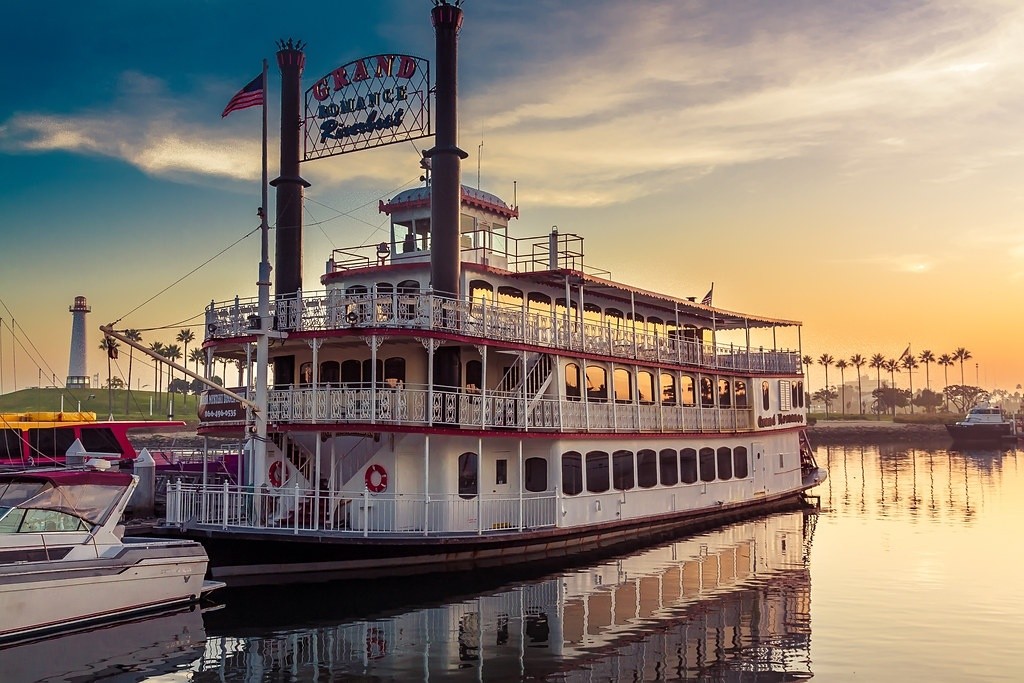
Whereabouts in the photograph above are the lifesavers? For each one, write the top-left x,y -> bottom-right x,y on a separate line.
364,464 -> 388,493
268,461 -> 289,488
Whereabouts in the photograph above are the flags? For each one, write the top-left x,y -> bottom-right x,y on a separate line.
222,72 -> 265,119
700,289 -> 712,304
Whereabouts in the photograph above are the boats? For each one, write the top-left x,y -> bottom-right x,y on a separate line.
189,507 -> 816,683
950,439 -> 1024,478
0,438 -> 226,650
99,0 -> 828,586
0,412 -> 244,536
944,401 -> 1024,439
